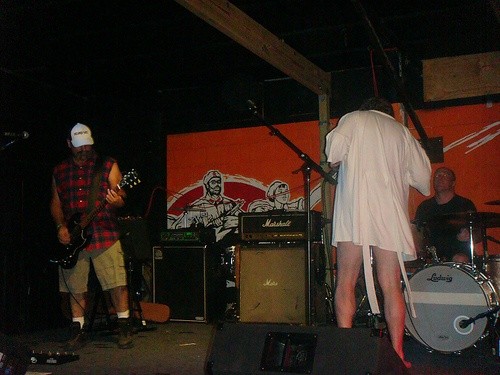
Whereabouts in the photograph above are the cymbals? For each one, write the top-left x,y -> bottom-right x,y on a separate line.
173,206 -> 206,212
439,209 -> 500,228
483,199 -> 500,206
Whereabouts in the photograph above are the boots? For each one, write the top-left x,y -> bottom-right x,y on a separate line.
117,316 -> 134,348
63,321 -> 89,350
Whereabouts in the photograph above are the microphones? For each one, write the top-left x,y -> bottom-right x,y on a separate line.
123,231 -> 130,236
3,131 -> 30,139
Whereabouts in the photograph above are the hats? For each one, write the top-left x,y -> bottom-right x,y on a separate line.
68,123 -> 94,148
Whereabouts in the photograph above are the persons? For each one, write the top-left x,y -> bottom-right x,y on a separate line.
50,122 -> 134,348
417,168 -> 476,265
325,97 -> 433,368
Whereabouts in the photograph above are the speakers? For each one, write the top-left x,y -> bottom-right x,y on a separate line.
314,327 -> 411,375
207,322 -> 316,375
153,245 -> 221,323
115,215 -> 155,259
238,210 -> 328,326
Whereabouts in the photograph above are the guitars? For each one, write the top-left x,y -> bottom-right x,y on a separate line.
56,169 -> 141,270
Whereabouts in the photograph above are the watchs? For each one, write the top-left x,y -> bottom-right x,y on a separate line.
56,224 -> 63,230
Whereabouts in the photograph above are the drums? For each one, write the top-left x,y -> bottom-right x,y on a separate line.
225,246 -> 236,274
403,260 -> 499,356
477,253 -> 500,297
403,218 -> 440,267
